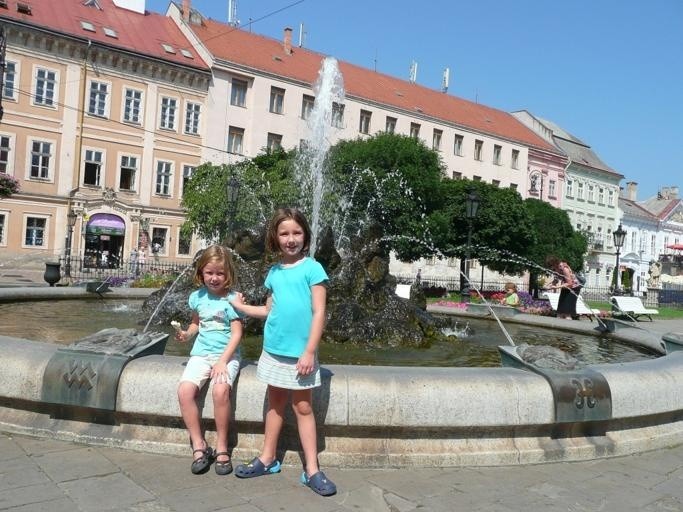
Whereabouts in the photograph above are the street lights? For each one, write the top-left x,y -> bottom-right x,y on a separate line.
226,172 -> 240,250
612,225 -> 627,313
65,210 -> 77,277
461,187 -> 481,303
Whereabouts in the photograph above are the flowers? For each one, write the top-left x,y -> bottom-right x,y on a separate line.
0,171 -> 21,199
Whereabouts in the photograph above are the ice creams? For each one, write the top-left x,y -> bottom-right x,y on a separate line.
170,320 -> 183,336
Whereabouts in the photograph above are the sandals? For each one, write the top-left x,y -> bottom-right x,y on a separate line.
213,449 -> 232,475
300,470 -> 336,496
235,457 -> 281,478
191,446 -> 212,473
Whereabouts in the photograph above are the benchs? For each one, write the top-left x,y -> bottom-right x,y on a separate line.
609,296 -> 659,321
543,292 -> 601,322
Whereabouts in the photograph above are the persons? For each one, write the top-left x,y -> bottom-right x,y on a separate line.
130,246 -> 145,272
227,207 -> 338,496
173,245 -> 245,476
500,282 -> 520,307
543,254 -> 583,320
